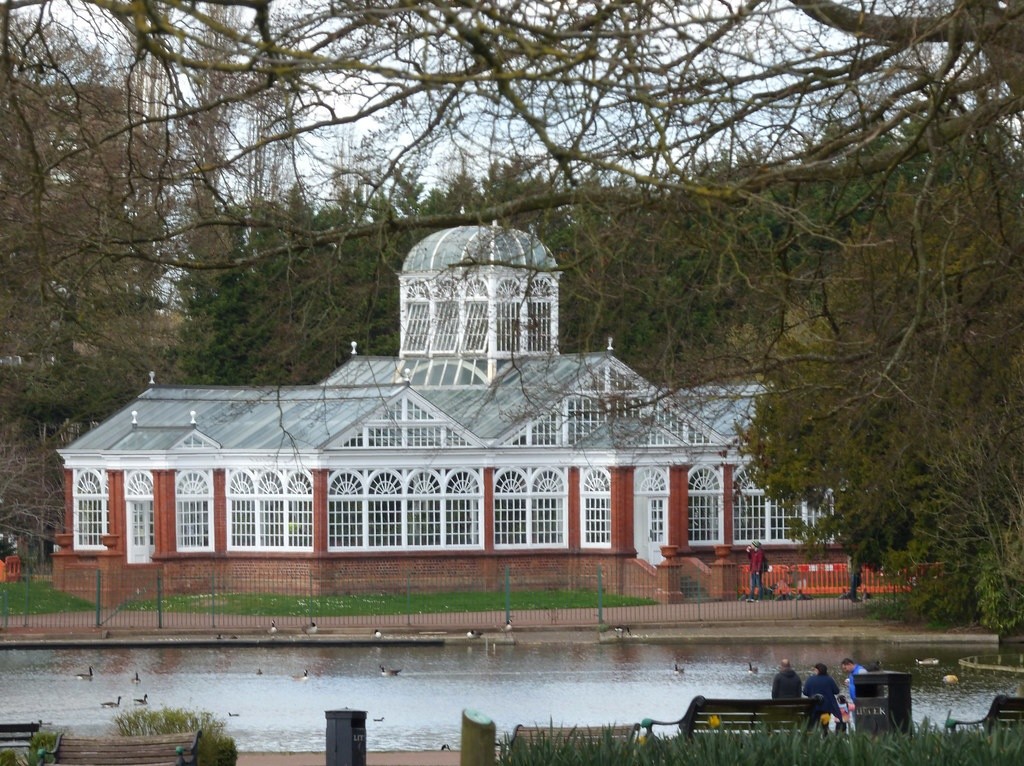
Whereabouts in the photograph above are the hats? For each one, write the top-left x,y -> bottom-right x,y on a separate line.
753,541 -> 762,548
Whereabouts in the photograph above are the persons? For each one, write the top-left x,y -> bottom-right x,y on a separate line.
772,660 -> 802,699
842,658 -> 868,725
803,664 -> 844,739
746,541 -> 764,602
834,695 -> 855,736
848,547 -> 862,602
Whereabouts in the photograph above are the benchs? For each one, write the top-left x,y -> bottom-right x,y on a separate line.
33,729 -> 202,766
0,720 -> 42,750
945,695 -> 1024,735
640,694 -> 824,737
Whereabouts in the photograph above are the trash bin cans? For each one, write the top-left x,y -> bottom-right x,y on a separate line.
851,671 -> 913,734
460,709 -> 496,765
325,709 -> 367,766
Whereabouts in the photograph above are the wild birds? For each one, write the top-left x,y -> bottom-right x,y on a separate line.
76,666 -> 93,677
379,664 -> 401,675
100,696 -> 121,708
132,672 -> 141,684
257,668 -> 263,674
374,629 -> 382,637
914,657 -> 939,663
134,694 -> 149,705
466,629 -> 483,638
500,620 -> 514,633
230,633 -> 239,639
441,744 -> 449,751
373,717 -> 386,721
216,634 -> 223,639
748,663 -> 759,672
228,712 -> 240,716
266,620 -> 277,634
291,669 -> 309,680
613,626 -> 633,637
673,664 -> 684,674
302,623 -> 317,634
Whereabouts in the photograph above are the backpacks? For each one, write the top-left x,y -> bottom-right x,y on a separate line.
762,557 -> 769,572
